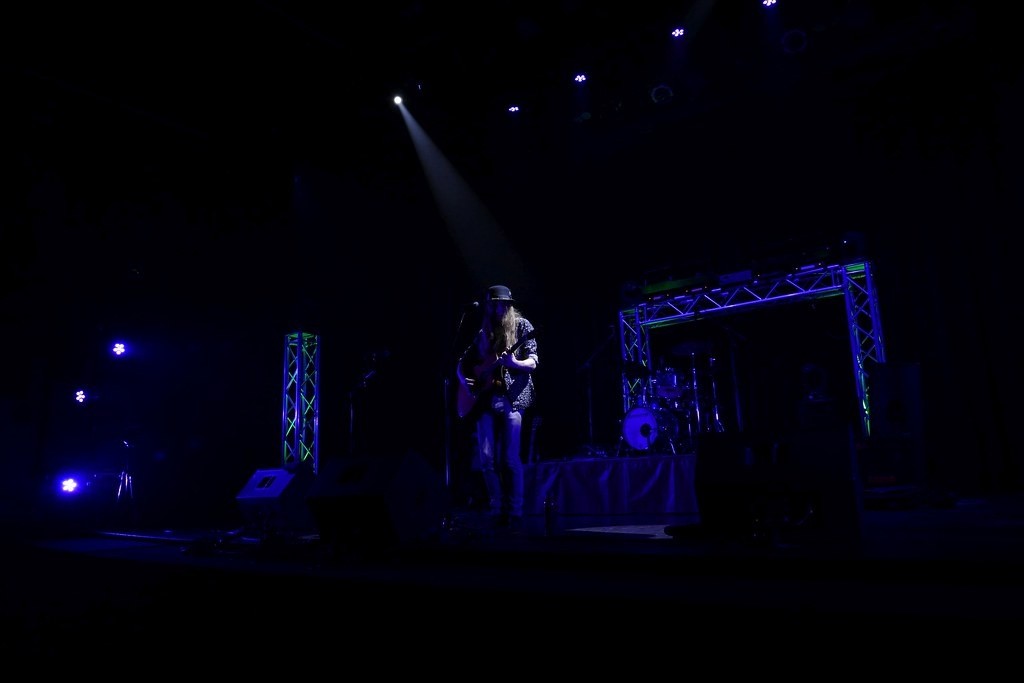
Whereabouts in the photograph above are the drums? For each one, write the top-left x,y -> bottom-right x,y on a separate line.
620,371 -> 685,452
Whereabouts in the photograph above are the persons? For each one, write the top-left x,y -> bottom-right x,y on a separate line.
456,286 -> 539,518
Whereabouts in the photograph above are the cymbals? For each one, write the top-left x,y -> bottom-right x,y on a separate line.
613,360 -> 646,377
669,353 -> 713,369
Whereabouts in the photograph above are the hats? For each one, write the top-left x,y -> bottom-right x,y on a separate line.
484,285 -> 514,302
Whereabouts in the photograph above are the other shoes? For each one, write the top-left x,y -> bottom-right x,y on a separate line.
507,497 -> 524,516
484,496 -> 503,515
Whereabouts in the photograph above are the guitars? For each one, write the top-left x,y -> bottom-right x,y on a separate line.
454,328 -> 542,421
